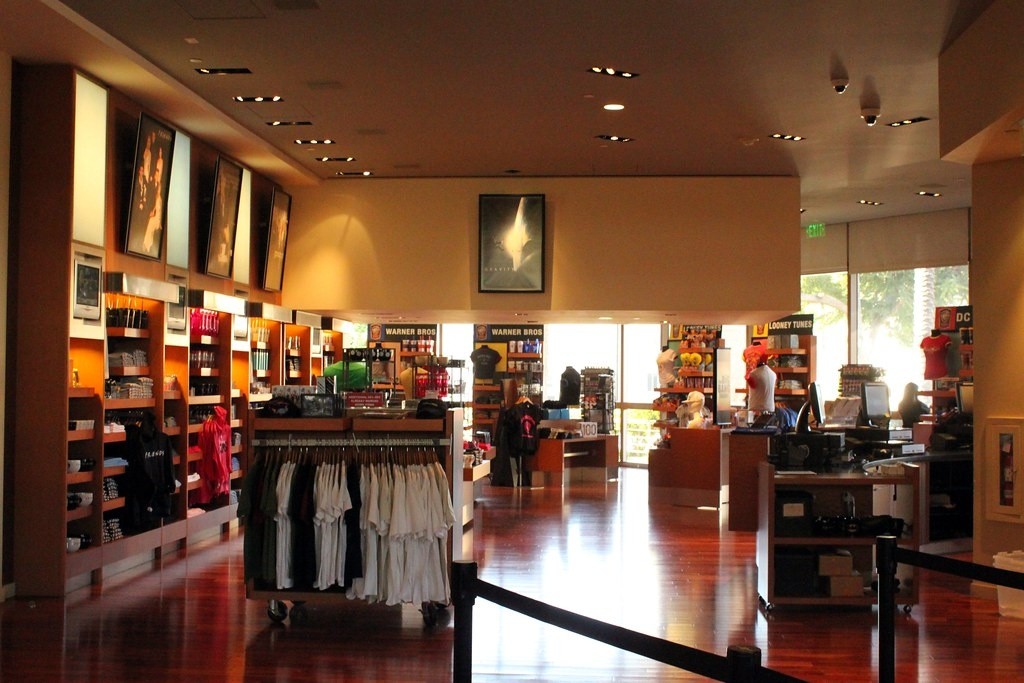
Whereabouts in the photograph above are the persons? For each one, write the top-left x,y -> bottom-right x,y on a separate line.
681,391 -> 705,428
743,341 -> 768,381
470,345 -> 502,379
559,366 -> 582,405
898,383 -> 931,429
920,328 -> 953,380
399,364 -> 431,400
745,361 -> 777,413
657,346 -> 678,384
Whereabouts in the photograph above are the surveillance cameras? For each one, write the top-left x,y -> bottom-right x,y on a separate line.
860,108 -> 881,125
833,79 -> 849,93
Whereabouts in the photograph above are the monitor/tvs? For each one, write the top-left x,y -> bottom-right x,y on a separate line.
955,382 -> 975,418
796,381 -> 823,434
855,382 -> 890,428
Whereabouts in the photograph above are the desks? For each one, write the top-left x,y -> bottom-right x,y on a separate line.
530,434 -> 619,474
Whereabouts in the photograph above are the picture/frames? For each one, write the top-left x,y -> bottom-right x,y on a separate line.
121,110 -> 176,262
200,154 -> 243,279
261,182 -> 292,292
479,193 -> 545,293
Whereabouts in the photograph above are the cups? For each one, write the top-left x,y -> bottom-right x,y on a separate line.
106,307 -> 149,329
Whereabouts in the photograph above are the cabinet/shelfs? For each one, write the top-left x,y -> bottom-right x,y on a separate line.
755,462 -> 922,609
472,352 -> 540,444
59,273 -> 343,589
656,346 -> 716,430
733,334 -> 816,412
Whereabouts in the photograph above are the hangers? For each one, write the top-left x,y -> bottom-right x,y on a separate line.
248,434 -> 441,469
775,400 -> 789,410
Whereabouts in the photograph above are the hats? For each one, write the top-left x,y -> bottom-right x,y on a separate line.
681,391 -> 705,404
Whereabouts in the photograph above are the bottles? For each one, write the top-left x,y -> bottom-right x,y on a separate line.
508,338 -> 542,371
401,335 -> 434,354
